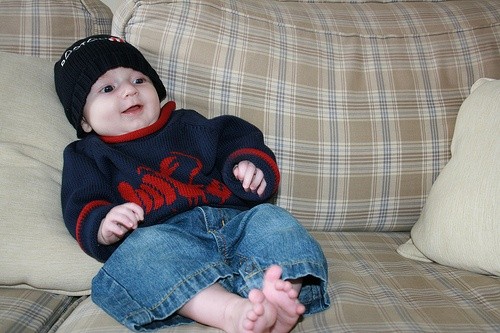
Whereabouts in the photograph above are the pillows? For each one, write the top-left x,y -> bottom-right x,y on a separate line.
394,77 -> 500,277
0,51 -> 104,297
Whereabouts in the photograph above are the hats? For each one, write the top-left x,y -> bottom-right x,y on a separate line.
54,34 -> 167,139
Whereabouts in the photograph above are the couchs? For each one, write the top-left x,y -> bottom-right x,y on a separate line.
0,0 -> 500,333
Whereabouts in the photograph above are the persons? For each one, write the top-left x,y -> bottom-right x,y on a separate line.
54,33 -> 332,333
